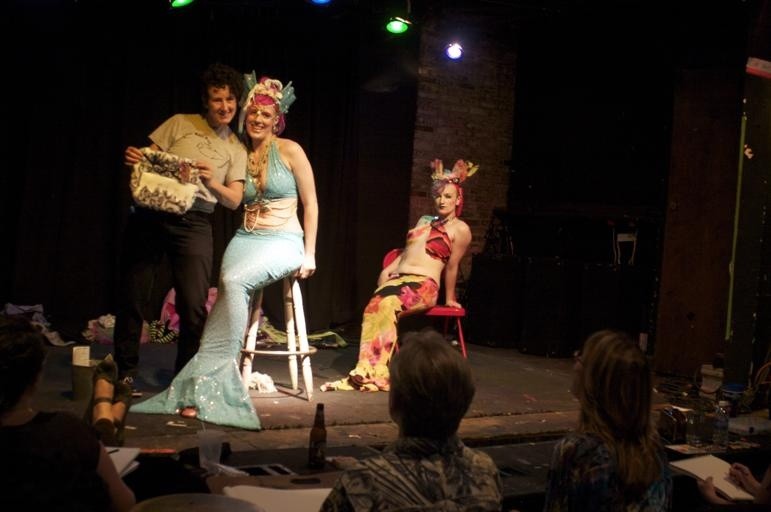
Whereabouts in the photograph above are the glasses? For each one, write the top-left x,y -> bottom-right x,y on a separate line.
572,350 -> 585,367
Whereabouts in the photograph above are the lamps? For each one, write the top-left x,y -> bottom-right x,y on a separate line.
381,1 -> 410,35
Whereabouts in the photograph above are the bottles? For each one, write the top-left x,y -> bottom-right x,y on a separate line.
309,404 -> 327,472
711,399 -> 728,450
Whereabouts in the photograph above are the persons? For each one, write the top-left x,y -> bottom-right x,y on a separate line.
0,314 -> 137,512
680,462 -> 770,511
113,68 -> 248,398
318,157 -> 481,393
126,68 -> 320,431
319,327 -> 506,512
542,327 -> 675,512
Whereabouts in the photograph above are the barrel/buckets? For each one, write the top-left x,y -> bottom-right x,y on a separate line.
698,361 -> 724,399
721,380 -> 747,419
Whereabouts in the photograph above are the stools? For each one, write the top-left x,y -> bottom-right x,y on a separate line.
239,266 -> 312,402
395,305 -> 467,365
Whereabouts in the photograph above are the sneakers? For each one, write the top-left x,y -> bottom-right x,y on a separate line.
117,378 -> 143,396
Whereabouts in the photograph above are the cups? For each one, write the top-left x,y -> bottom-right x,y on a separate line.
196,429 -> 225,471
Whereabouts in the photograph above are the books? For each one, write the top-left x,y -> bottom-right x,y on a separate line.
667,453 -> 758,503
104,446 -> 141,479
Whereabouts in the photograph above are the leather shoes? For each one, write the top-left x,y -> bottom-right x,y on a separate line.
104,382 -> 131,446
181,407 -> 197,417
89,360 -> 117,424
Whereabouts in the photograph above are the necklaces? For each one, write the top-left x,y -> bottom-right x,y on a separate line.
248,140 -> 270,174
